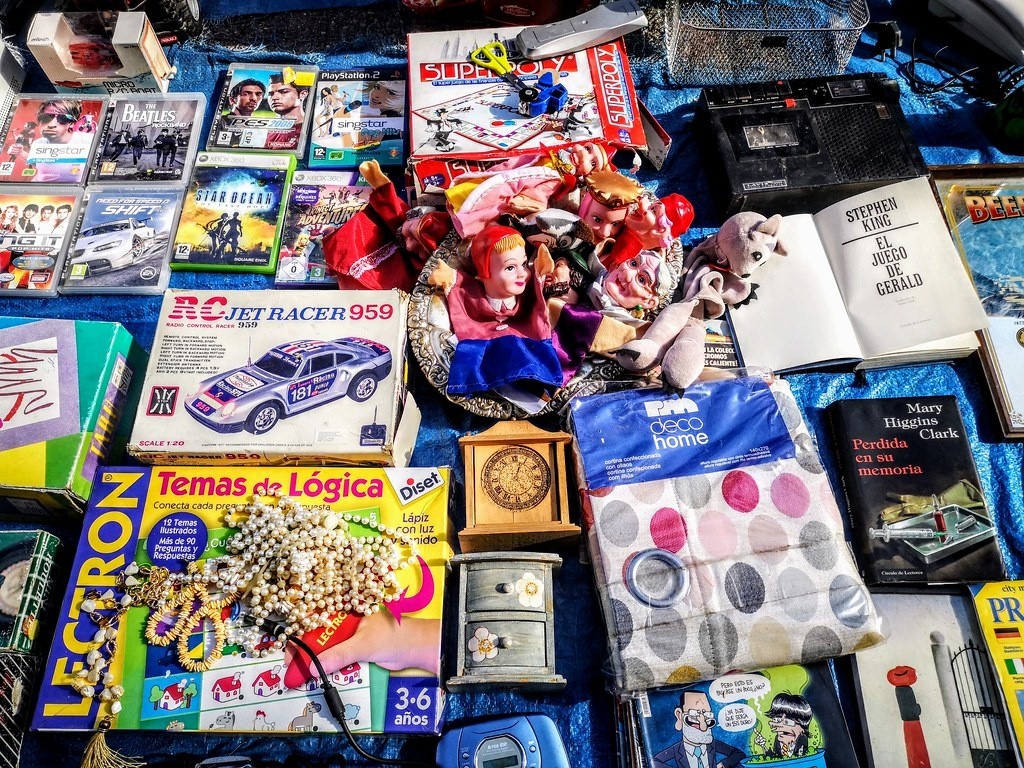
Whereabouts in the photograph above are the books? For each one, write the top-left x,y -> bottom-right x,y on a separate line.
968,580 -> 1024,768
0,530 -> 65,768
613,660 -> 859,768
724,177 -> 991,372
825,395 -> 1008,594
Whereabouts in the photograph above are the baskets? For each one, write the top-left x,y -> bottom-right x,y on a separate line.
663,0 -> 870,86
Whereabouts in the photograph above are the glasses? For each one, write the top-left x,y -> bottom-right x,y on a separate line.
38,113 -> 77,125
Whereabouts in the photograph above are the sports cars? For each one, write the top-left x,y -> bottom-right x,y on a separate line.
184,334 -> 393,436
66,217 -> 158,280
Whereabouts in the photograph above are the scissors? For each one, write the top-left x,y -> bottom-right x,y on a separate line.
471,42 -> 527,90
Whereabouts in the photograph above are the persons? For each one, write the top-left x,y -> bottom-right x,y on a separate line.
6,100 -> 98,183
284,607 -> 442,678
0,204 -> 73,287
321,141 -> 693,415
215,74 -> 310,150
108,129 -> 182,167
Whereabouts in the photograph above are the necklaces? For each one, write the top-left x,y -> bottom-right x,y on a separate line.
70,489 -> 417,768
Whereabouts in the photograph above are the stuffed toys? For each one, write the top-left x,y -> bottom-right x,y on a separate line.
614,212 -> 789,386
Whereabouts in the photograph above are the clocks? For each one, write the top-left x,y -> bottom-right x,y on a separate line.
456,418 -> 582,553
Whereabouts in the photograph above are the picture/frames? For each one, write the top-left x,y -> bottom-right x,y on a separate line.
844,582 -> 1018,768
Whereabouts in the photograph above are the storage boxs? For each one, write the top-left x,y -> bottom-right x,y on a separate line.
25,11 -> 171,95
405,24 -> 673,208
126,287 -> 422,469
621,659 -> 861,768
30,463 -> 467,739
0,527 -> 67,656
0,314 -> 148,524
